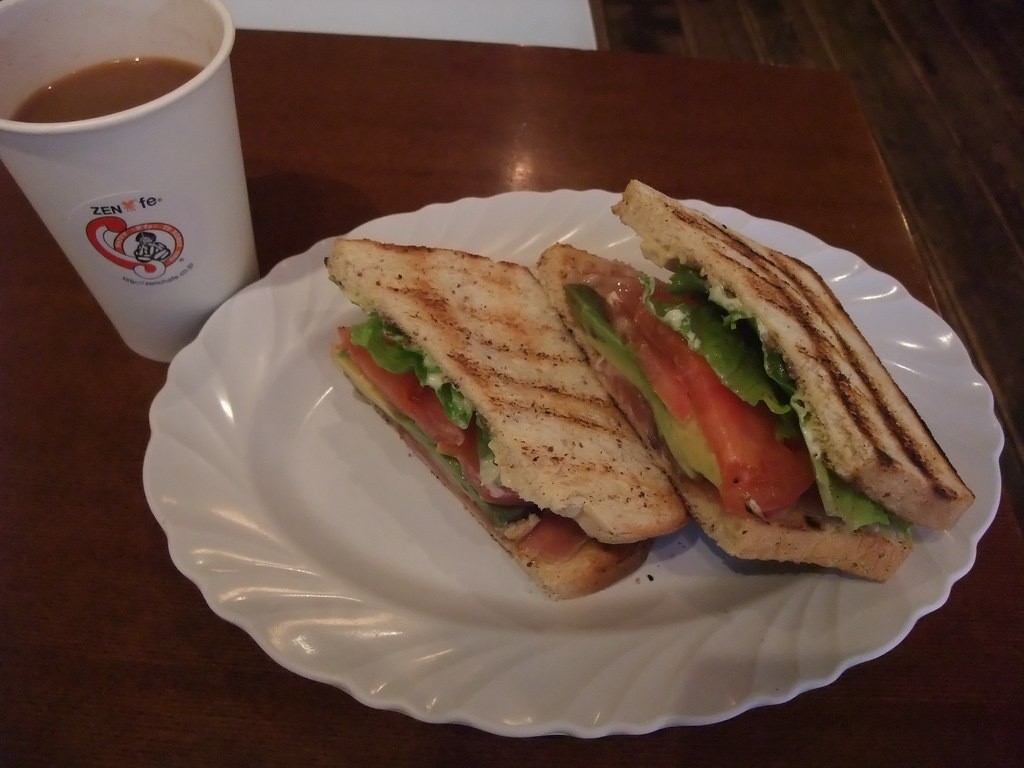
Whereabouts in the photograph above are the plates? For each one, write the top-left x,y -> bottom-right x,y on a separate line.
142,188 -> 1007,741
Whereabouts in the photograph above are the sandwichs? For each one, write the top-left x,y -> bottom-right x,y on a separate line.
325,177 -> 975,605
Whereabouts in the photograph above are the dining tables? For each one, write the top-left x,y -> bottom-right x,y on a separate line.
0,28 -> 1024,766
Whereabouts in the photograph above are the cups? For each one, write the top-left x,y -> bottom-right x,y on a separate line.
0,0 -> 261,363
